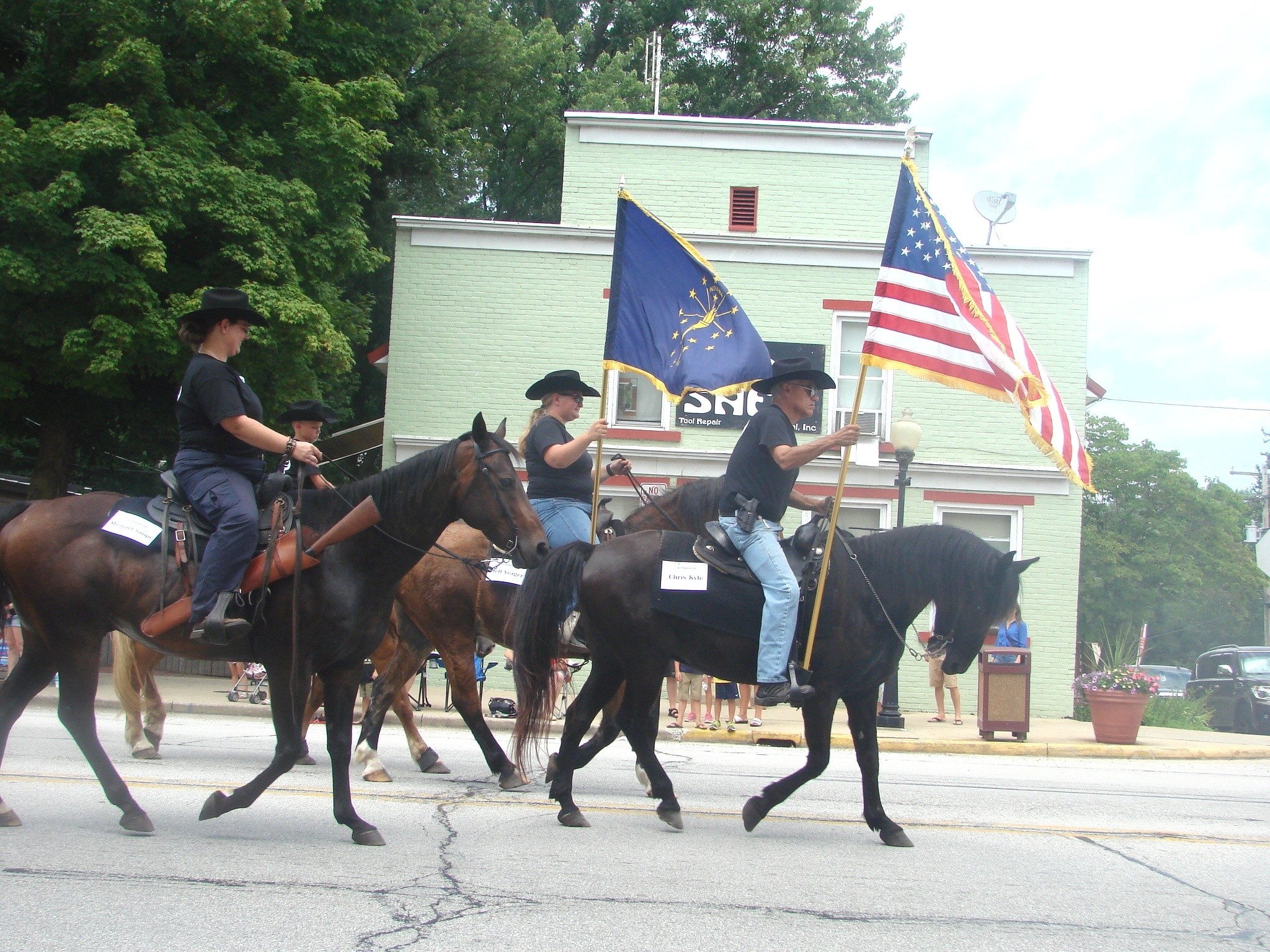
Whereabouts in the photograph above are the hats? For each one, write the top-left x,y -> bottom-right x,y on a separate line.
751,357 -> 836,393
175,288 -> 270,328
525,370 -> 601,400
277,400 -> 339,424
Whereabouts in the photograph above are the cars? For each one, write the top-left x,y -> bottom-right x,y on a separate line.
1120,665 -> 1191,698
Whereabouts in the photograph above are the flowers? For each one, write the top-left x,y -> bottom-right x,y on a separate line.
1071,619 -> 1160,699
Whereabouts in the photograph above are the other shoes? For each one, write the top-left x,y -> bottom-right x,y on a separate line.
262,699 -> 271,705
226,691 -> 249,699
543,712 -> 556,721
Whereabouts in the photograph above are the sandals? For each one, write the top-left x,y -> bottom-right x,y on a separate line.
352,719 -> 364,726
668,708 -> 679,718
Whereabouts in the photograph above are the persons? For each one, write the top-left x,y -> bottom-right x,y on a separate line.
505,649 -> 571,720
719,358 -> 860,707
261,683 -> 271,705
352,657 -> 378,724
991,601 -> 1027,664
276,399 -> 337,491
3,603 -> 23,680
172,288 -> 323,645
228,661 -> 249,699
925,599 -> 963,725
308,672 -> 326,723
519,370 -> 632,653
664,660 -> 762,731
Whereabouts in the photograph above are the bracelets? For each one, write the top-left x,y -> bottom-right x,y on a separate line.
285,436 -> 296,458
607,463 -> 615,476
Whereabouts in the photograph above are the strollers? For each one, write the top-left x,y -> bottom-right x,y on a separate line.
227,662 -> 269,704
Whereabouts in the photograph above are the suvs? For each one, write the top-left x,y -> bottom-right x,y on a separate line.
1185,644 -> 1270,736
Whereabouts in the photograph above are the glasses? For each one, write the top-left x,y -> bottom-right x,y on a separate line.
558,393 -> 583,404
789,381 -> 816,397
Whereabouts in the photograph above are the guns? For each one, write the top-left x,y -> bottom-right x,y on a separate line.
304,495 -> 384,558
733,493 -> 761,512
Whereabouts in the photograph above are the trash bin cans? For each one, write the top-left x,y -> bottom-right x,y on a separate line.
976,646 -> 1031,742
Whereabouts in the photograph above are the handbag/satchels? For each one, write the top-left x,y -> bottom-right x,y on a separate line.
488,695 -> 518,718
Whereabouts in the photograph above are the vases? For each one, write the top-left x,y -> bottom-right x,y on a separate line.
1083,689 -> 1150,744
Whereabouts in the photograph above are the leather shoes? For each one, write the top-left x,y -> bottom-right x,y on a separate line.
558,628 -> 589,653
190,615 -> 252,645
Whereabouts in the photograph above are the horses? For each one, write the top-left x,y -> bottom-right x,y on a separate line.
0,413 -> 1041,848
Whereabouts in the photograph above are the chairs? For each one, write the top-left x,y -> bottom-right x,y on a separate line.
503,657 -> 582,719
407,652 -> 497,712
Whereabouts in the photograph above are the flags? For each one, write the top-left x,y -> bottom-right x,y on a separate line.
603,174 -> 773,406
861,127 -> 1100,496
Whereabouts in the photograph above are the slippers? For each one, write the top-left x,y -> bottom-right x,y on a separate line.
953,720 -> 963,725
750,718 -> 762,727
667,723 -> 683,728
928,718 -> 946,722
695,723 -> 707,730
725,715 -> 748,724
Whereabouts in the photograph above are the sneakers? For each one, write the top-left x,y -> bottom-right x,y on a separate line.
755,681 -> 815,706
709,720 -> 721,730
309,714 -> 327,725
685,712 -> 697,722
727,722 -> 736,732
704,713 -> 714,724
